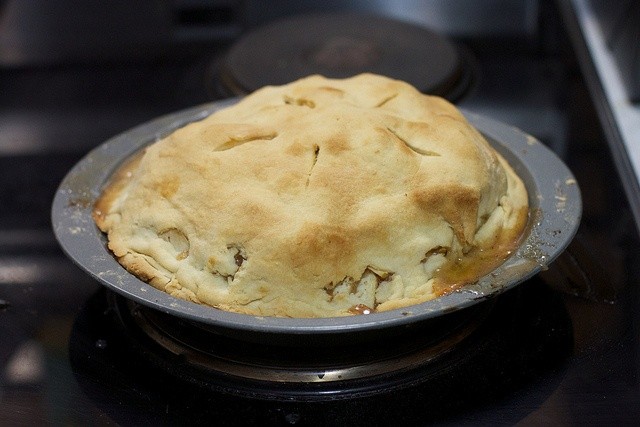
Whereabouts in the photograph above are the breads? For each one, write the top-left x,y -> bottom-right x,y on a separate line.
93,73 -> 529,318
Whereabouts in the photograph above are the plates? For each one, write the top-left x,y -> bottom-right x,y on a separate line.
50,91 -> 583,334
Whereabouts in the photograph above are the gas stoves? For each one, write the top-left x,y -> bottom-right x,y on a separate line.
67,0 -> 640,426
